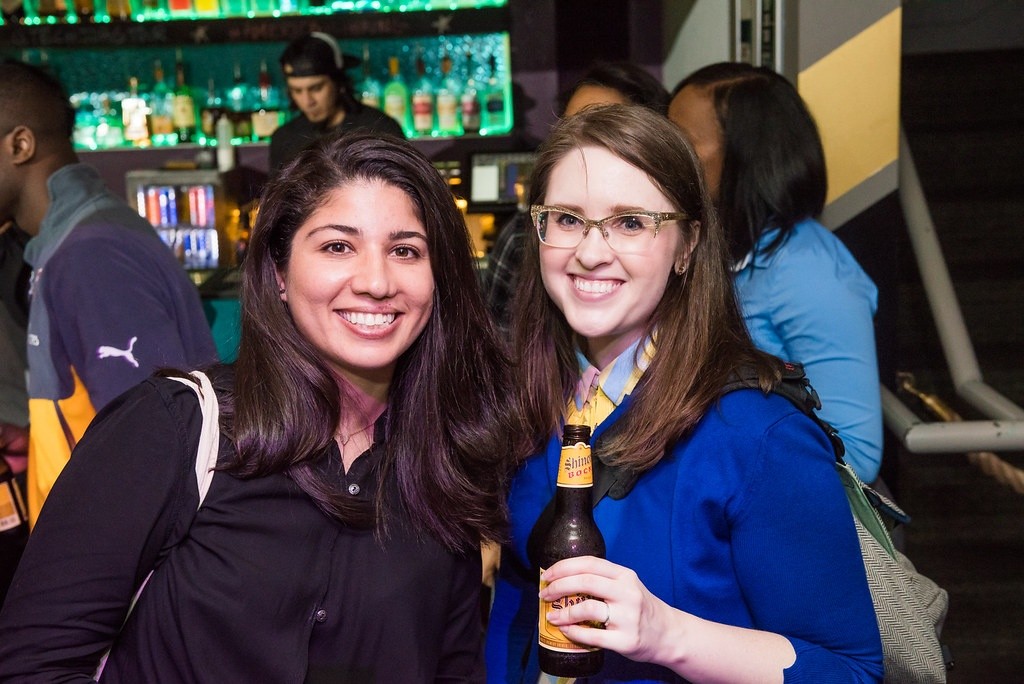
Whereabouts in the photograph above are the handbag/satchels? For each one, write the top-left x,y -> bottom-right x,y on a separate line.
836,462 -> 949,684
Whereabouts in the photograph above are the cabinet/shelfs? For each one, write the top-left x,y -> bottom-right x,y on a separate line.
0,0 -> 561,171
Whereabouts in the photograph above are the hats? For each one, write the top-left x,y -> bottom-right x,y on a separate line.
280,32 -> 360,76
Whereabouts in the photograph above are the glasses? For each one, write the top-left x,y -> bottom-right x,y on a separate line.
530,204 -> 689,254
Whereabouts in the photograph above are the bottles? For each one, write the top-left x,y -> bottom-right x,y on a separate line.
0,1 -> 509,26
533,424 -> 608,677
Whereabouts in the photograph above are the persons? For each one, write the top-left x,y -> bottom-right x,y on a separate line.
267,30 -> 408,166
479,63 -> 673,321
664,62 -> 886,487
0,47 -> 222,542
0,125 -> 529,684
476,99 -> 887,684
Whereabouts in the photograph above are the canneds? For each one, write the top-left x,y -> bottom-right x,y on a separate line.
137,183 -> 220,269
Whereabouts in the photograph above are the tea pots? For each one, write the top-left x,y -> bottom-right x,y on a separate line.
62,48 -> 508,156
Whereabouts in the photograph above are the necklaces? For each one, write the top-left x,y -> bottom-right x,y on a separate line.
335,422 -> 374,446
